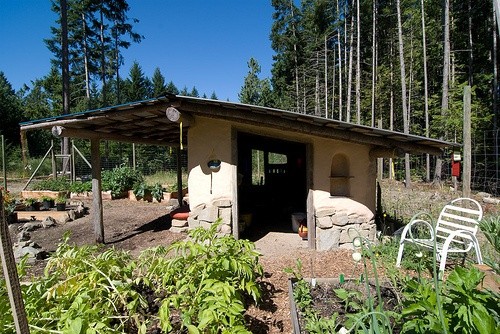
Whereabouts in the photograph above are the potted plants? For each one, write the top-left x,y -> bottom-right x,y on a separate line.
23,198 -> 40,211
5,203 -> 18,225
55,194 -> 67,211
37,194 -> 53,210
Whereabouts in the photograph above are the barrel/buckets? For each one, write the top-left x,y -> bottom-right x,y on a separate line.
291,211 -> 306,233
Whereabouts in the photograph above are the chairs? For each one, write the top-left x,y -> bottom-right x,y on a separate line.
396,197 -> 484,280
